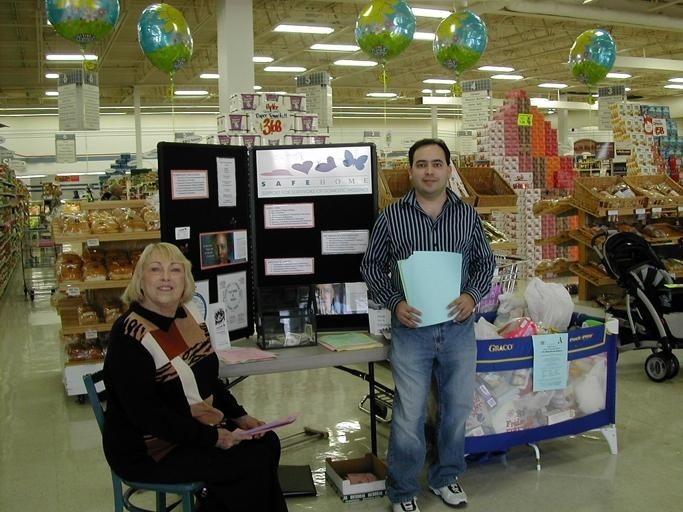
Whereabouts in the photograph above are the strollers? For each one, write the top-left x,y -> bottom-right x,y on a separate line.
590,230 -> 683,382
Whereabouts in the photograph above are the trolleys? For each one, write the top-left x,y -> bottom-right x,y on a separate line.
20,229 -> 58,301
357,250 -> 534,424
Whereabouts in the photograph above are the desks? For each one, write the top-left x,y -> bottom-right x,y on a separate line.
218,333 -> 389,457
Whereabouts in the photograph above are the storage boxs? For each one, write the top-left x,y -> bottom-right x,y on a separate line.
217,93 -> 329,145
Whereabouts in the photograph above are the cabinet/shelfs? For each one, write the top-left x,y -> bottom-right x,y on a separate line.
378,166 -> 518,264
0,162 -> 161,405
533,152 -> 683,308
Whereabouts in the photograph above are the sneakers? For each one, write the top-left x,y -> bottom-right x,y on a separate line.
428,481 -> 468,507
393,497 -> 419,512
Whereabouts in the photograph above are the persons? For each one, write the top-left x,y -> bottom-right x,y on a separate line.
71,183 -> 124,201
317,284 -> 351,315
213,233 -> 236,265
102,242 -> 288,512
360,139 -> 496,512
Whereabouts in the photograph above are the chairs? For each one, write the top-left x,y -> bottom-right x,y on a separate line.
82,372 -> 204,512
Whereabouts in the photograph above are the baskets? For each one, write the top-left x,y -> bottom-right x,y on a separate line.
576,173 -> 683,209
379,168 -> 412,208
448,167 -> 517,207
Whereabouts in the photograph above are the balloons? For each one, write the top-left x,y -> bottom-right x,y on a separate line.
433,7 -> 490,81
355,1 -> 416,65
44,0 -> 120,50
568,27 -> 617,89
137,2 -> 194,75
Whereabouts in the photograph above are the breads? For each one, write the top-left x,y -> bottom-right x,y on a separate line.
530,175 -> 682,308
47,205 -> 160,364
